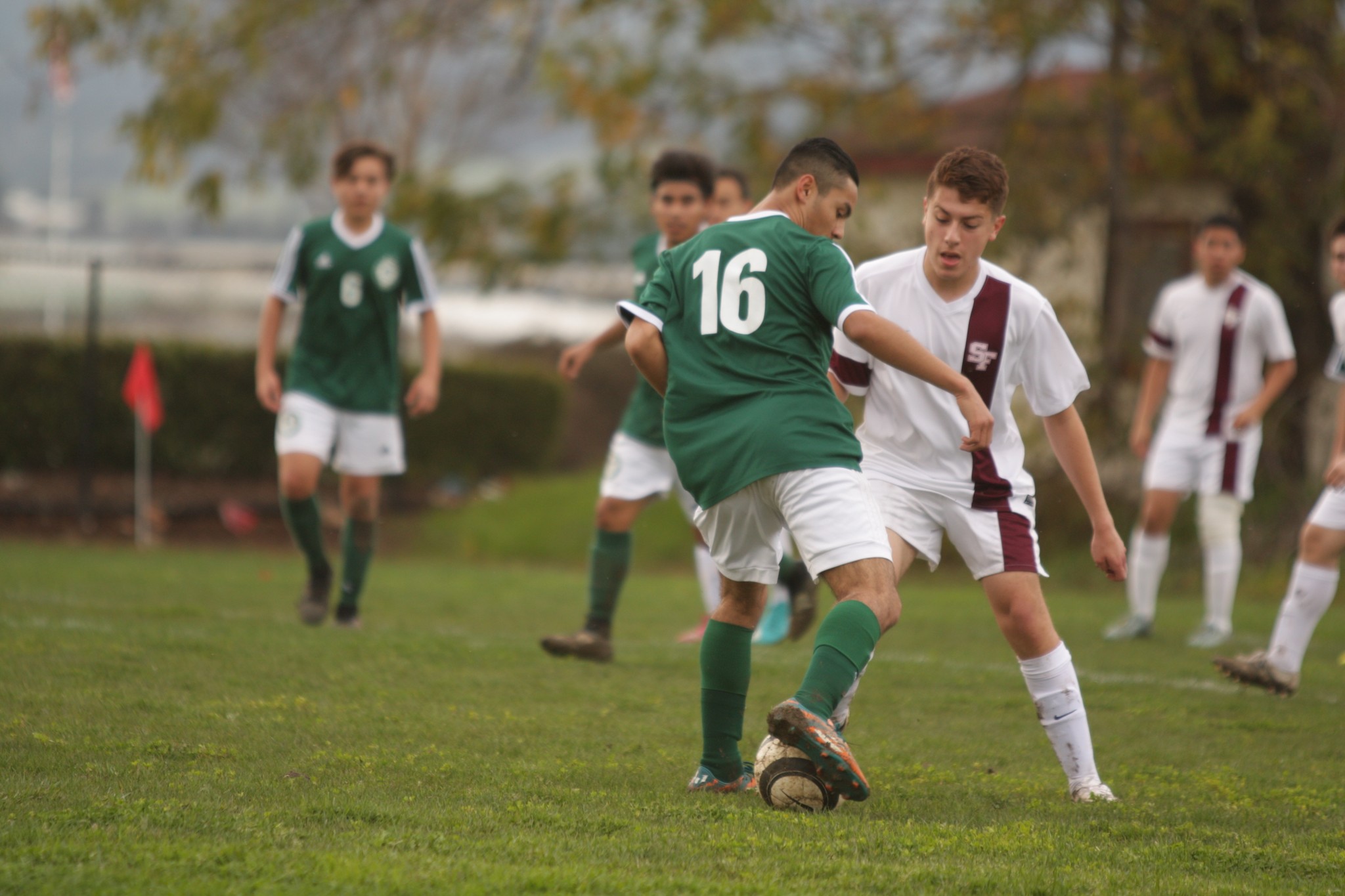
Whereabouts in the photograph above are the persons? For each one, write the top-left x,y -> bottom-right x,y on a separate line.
828,146 -> 1128,806
539,152 -> 819,665
617,137 -> 993,801
1106,216 -> 1299,649
1211,222 -> 1345,697
254,142 -> 441,632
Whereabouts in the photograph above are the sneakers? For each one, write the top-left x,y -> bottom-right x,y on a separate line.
540,631 -> 613,661
1212,651 -> 1300,696
788,561 -> 819,639
766,696 -> 872,801
688,761 -> 758,795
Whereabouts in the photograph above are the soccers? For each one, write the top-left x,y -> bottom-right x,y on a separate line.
757,736 -> 840,811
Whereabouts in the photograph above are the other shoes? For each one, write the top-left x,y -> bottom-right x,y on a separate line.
1072,785 -> 1116,803
834,711 -> 849,732
750,604 -> 790,644
334,601 -> 356,623
299,564 -> 332,625
1108,617 -> 1155,641
676,617 -> 709,642
1189,627 -> 1231,650
